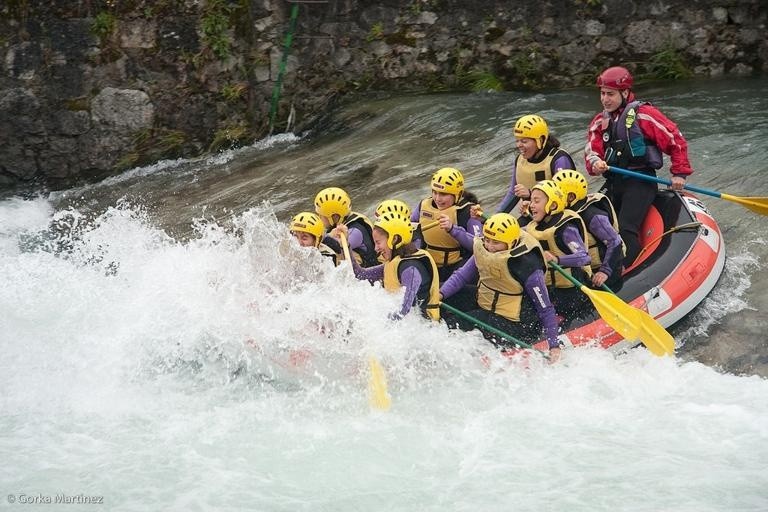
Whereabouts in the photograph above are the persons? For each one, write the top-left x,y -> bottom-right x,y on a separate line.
313,185 -> 378,269
488,113 -> 578,228
516,178 -> 594,326
332,210 -> 442,330
430,210 -> 563,367
582,65 -> 694,270
548,167 -> 626,296
286,211 -> 338,284
373,198 -> 413,267
405,168 -> 486,287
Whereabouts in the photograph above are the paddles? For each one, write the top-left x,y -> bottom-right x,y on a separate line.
341,230 -> 392,411
590,271 -> 676,360
551,263 -> 644,342
606,164 -> 768,216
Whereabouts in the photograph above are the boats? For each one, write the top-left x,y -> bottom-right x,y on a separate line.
264,185 -> 729,402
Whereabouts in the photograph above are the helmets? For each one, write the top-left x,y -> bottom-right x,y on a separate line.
516,114 -> 549,150
432,168 -> 465,204
531,169 -> 588,216
596,67 -> 634,90
484,213 -> 519,250
375,200 -> 412,250
290,187 -> 350,247
